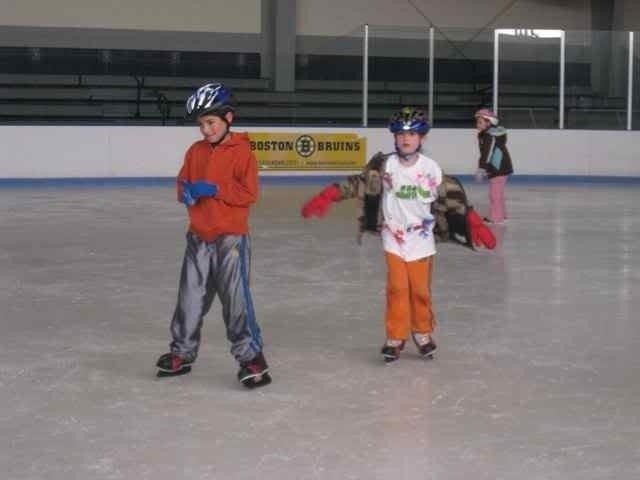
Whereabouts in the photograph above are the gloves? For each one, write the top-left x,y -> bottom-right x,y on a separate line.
303,186 -> 339,218
466,207 -> 496,249
183,179 -> 216,207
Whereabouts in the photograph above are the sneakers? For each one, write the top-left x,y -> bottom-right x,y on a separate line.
156,353 -> 193,372
239,352 -> 269,382
381,340 -> 404,358
412,332 -> 437,356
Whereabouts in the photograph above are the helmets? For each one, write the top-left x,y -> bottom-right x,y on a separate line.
475,108 -> 499,127
389,107 -> 430,133
185,82 -> 238,120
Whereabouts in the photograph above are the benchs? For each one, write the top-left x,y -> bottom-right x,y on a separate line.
0,73 -> 636,130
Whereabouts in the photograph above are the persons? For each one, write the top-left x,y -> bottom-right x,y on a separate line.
157,82 -> 273,386
301,108 -> 498,362
474,107 -> 515,223
